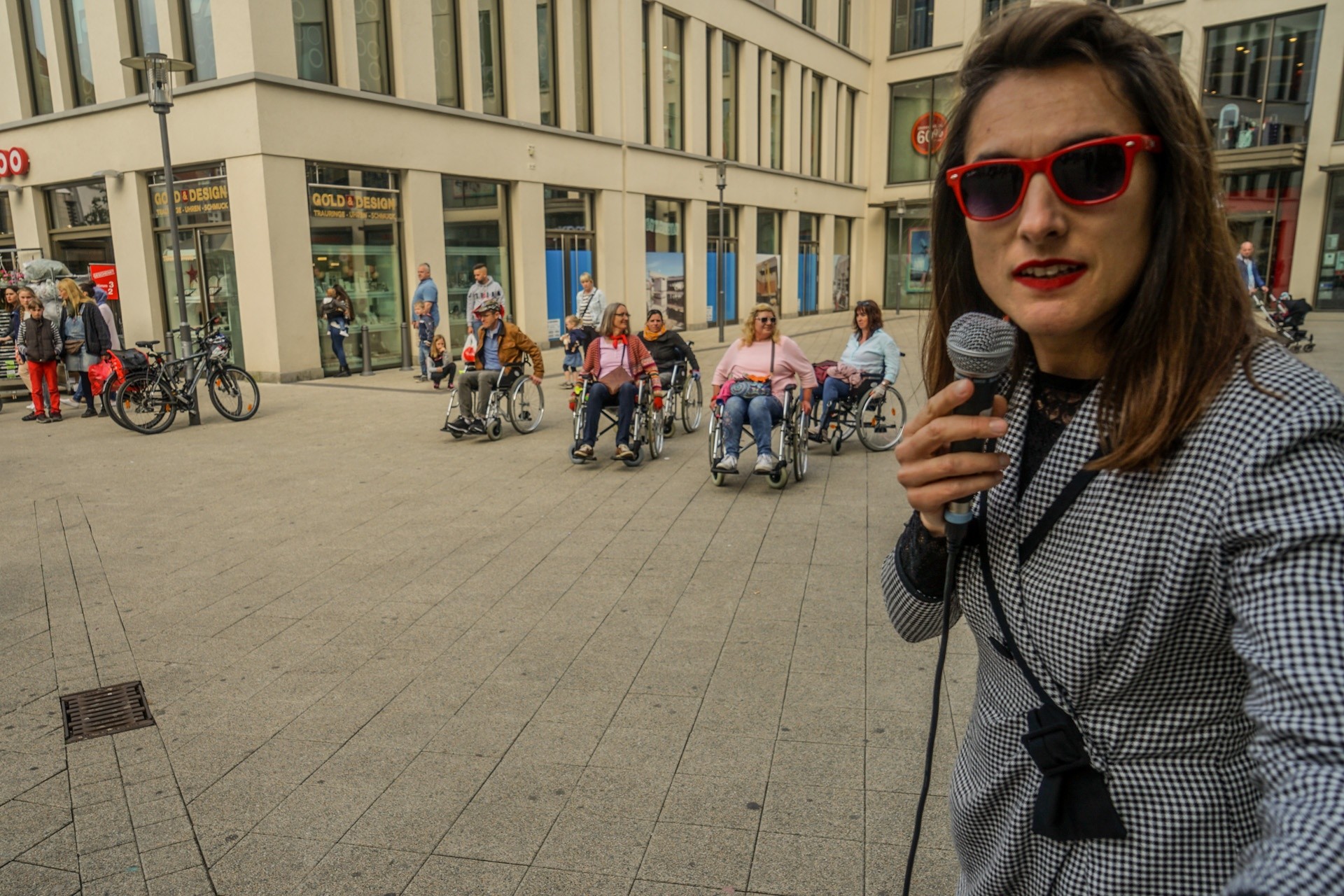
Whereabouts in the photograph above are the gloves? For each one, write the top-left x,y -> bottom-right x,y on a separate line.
653,396 -> 663,413
568,391 -> 578,412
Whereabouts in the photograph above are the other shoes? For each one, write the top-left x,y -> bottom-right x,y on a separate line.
790,422 -> 827,442
433,384 -> 441,391
447,384 -> 457,389
413,374 -> 429,383
21,387 -> 119,423
340,330 -> 349,337
558,381 -> 573,388
328,331 -> 333,336
338,372 -> 350,376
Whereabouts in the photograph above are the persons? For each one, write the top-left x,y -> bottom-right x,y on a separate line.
1265,291 -> 1290,322
709,303 -> 819,473
411,262 -> 441,334
318,284 -> 355,377
446,299 -> 545,433
57,278 -> 112,417
637,309 -> 701,391
414,301 -> 434,383
574,302 -> 663,457
576,272 -> 606,362
800,300 -> 900,442
558,315 -> 583,388
16,298 -> 63,423
0,286 -> 48,410
1236,241 -> 1269,296
877,5 -> 1344,896
60,281 -> 121,408
466,263 -> 506,347
427,334 -> 456,391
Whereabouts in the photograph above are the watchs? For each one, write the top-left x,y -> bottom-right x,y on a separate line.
882,381 -> 888,389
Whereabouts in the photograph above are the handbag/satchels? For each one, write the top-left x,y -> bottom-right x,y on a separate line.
731,378 -> 772,400
64,339 -> 85,353
89,348 -> 151,395
460,333 -> 479,366
598,367 -> 635,395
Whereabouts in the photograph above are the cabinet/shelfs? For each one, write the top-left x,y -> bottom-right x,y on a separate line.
310,245 -> 404,371
445,247 -> 502,356
162,248 -> 244,370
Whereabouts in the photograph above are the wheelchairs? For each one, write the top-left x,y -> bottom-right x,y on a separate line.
812,352 -> 908,455
568,340 -> 704,469
439,352 -> 547,443
708,384 -> 809,489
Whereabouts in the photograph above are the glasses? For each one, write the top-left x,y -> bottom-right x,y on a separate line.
756,316 -> 777,324
946,134 -> 1158,220
858,301 -> 871,309
614,312 -> 631,317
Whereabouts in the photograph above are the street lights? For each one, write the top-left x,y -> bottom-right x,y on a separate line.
896,197 -> 907,315
119,53 -> 203,427
703,160 -> 731,343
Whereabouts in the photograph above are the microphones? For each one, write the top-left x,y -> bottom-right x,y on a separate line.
942,310 -> 1015,544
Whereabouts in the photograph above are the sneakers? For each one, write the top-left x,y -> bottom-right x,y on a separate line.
446,416 -> 486,433
574,443 -> 593,459
615,444 -> 634,456
753,454 -> 773,475
716,454 -> 737,470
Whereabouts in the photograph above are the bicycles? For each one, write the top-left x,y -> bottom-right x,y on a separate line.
100,312 -> 260,435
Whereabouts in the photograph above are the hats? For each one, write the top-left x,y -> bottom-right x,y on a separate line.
472,301 -> 501,313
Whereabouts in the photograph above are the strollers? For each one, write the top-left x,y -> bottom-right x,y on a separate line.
1251,290 -> 1316,354
